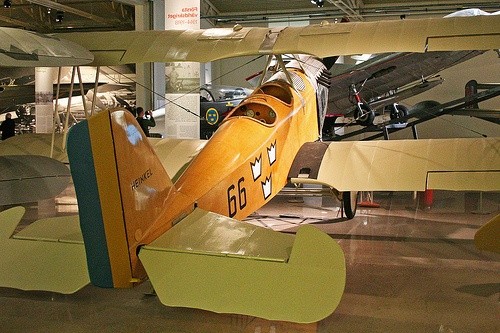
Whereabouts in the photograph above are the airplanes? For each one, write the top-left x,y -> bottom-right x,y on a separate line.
0,13 -> 500,323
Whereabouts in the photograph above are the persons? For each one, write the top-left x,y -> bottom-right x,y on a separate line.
116,101 -> 134,109
168,68 -> 178,87
135,107 -> 156,136
0,113 -> 15,140
